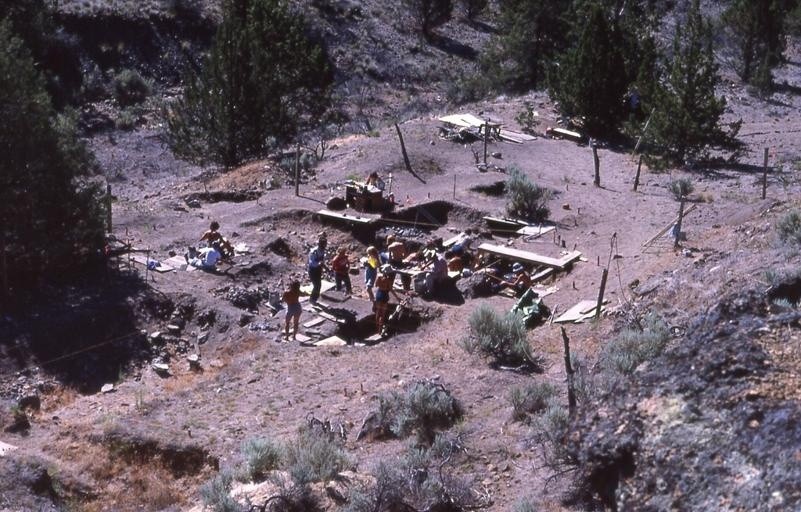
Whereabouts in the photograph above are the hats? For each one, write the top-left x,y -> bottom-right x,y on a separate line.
512,263 -> 523,272
381,263 -> 396,274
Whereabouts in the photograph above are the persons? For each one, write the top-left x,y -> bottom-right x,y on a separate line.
283,280 -> 312,340
423,228 -> 477,298
363,246 -> 381,301
309,237 -> 327,305
500,263 -> 530,295
373,264 -> 397,335
365,171 -> 385,190
387,235 -> 407,262
198,221 -> 230,270
332,247 -> 351,293
